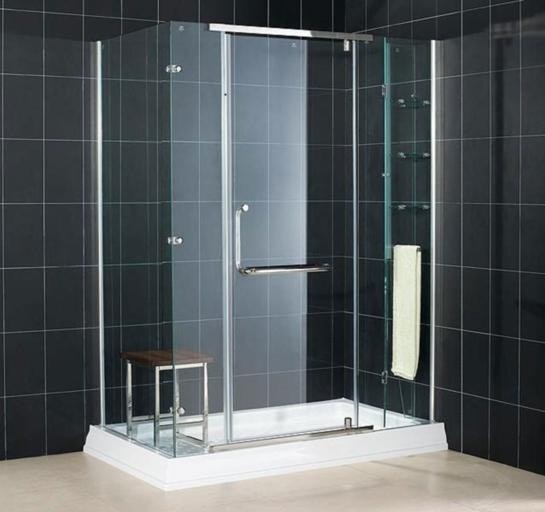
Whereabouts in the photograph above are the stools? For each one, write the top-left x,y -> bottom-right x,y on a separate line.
119,349 -> 216,448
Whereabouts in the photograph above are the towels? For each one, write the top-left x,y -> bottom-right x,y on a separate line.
390,243 -> 421,381
